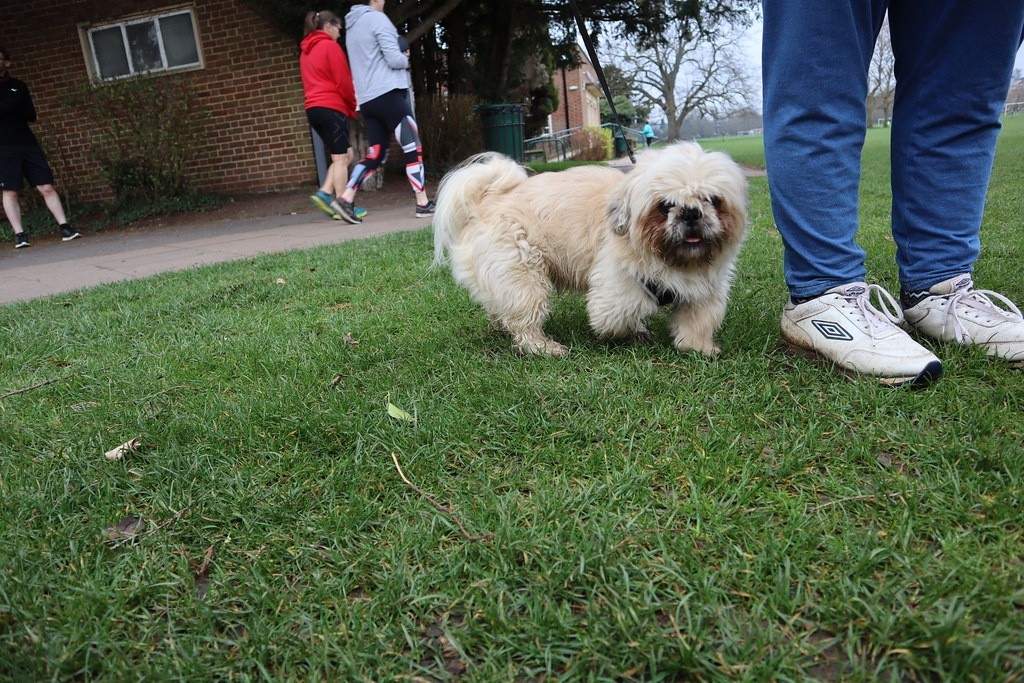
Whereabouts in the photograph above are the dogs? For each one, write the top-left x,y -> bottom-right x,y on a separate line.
429,135 -> 747,359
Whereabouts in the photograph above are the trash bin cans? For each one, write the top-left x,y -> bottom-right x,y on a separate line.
474,102 -> 525,164
616,137 -> 628,152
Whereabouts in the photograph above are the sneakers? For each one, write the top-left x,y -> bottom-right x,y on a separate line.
899,273 -> 1024,363
332,206 -> 368,220
309,190 -> 335,216
14,231 -> 30,248
62,226 -> 81,241
415,200 -> 435,218
330,197 -> 362,224
780,282 -> 943,391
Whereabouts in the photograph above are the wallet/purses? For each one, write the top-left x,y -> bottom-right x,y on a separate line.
398,35 -> 410,52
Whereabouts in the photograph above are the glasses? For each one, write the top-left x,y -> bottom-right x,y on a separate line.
331,23 -> 343,35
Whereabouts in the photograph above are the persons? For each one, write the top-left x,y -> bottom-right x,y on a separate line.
0,44 -> 81,248
763,0 -> 1024,387
639,121 -> 654,146
300,0 -> 435,222
